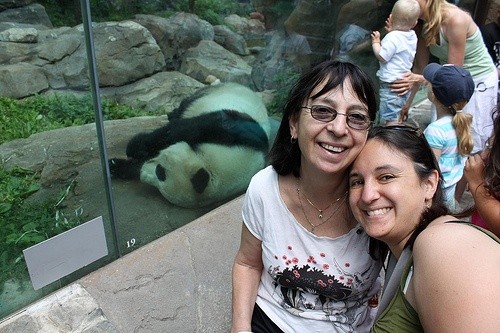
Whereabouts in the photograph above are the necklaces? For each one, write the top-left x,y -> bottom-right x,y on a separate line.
296,176 -> 349,231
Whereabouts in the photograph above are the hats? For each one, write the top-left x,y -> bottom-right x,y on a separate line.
422,63 -> 475,110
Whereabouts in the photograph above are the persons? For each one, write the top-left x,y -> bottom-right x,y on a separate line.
372,0 -> 500,238
249,0 -> 384,105
231,58 -> 389,333
348,122 -> 500,333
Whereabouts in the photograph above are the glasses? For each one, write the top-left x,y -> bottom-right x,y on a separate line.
297,105 -> 375,130
373,125 -> 426,143
